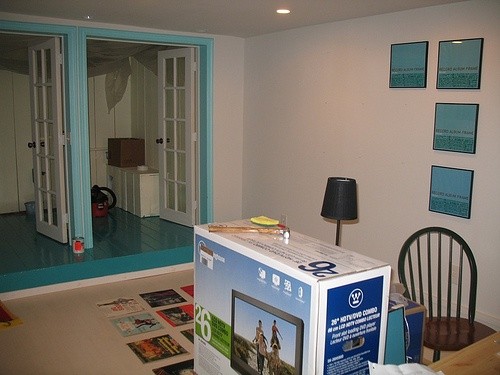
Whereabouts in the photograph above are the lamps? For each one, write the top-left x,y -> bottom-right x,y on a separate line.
320,176 -> 358,246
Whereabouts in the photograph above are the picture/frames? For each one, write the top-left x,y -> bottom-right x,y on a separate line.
428,165 -> 474,219
389,41 -> 429,88
438,38 -> 484,89
433,101 -> 479,154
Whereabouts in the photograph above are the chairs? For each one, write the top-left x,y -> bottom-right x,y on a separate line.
399,226 -> 497,363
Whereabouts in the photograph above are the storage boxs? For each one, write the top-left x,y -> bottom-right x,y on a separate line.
384,297 -> 426,367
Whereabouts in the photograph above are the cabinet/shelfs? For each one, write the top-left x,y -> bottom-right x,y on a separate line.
107,164 -> 161,218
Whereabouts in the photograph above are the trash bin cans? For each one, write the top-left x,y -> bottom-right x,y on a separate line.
25,200 -> 36,223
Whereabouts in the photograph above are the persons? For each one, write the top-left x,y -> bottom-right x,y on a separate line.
253,319 -> 284,375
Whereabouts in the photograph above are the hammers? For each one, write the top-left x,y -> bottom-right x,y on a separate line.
209,226 -> 290,240
